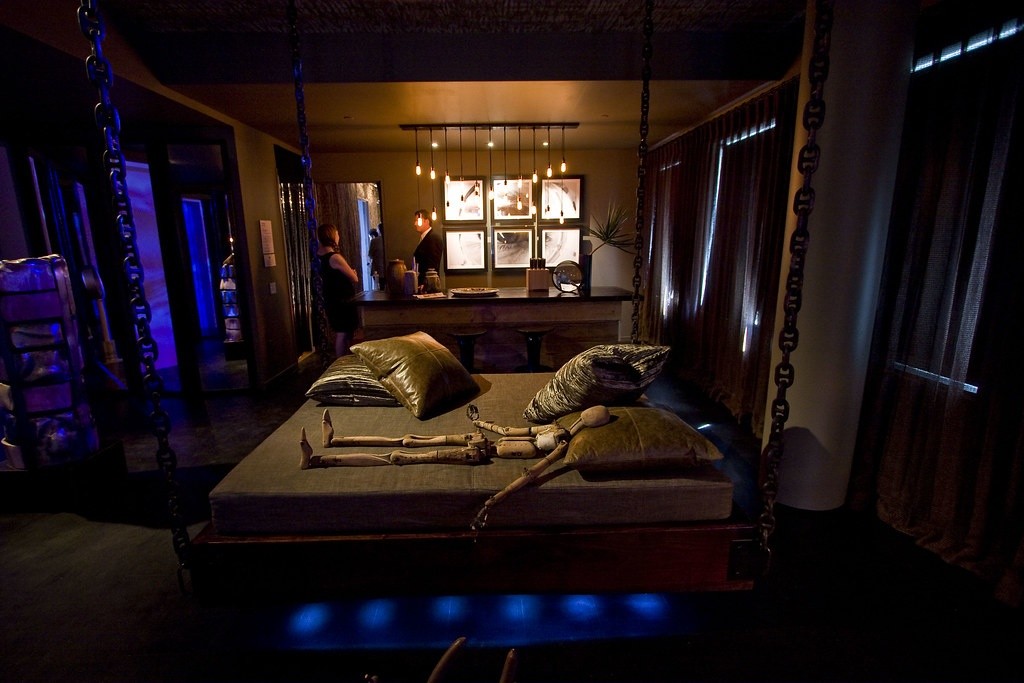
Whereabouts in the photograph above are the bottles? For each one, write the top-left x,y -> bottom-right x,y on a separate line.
404,270 -> 418,295
423,268 -> 442,293
386,259 -> 408,295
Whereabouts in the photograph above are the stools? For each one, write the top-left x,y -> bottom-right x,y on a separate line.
447,328 -> 486,374
516,325 -> 553,372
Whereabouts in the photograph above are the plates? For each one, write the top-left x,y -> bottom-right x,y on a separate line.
450,287 -> 499,297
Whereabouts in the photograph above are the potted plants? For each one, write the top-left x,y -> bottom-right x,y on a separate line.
579,202 -> 636,295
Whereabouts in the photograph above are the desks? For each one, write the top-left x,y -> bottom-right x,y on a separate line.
353,287 -> 643,365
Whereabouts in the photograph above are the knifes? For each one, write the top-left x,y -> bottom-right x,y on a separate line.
530,257 -> 545,270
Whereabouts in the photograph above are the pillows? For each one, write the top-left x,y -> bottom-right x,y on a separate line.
521,344 -> 673,423
556,404 -> 725,473
305,352 -> 398,407
349,332 -> 481,418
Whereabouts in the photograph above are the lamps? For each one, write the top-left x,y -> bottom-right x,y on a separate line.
398,123 -> 578,229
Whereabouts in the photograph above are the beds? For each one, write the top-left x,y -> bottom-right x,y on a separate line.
176,373 -> 771,597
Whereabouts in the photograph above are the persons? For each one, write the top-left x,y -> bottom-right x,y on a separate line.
368,223 -> 385,290
299,406 -> 609,529
412,209 -> 443,290
315,223 -> 360,355
